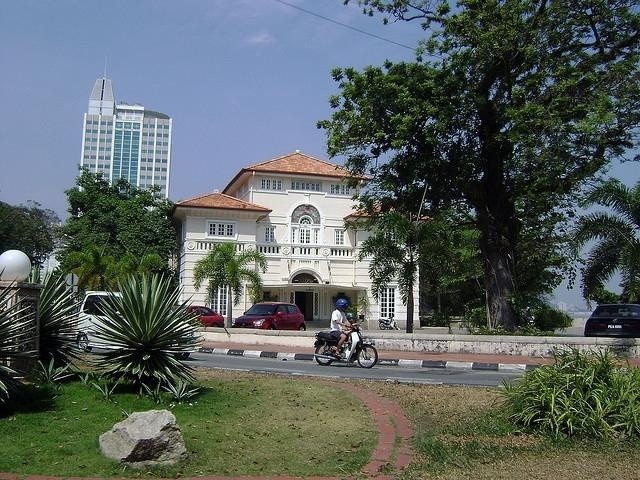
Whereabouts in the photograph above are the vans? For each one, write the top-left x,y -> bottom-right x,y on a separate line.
75,291 -> 196,362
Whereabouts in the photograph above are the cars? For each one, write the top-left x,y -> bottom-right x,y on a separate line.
185,306 -> 224,328
582,303 -> 639,340
235,300 -> 306,331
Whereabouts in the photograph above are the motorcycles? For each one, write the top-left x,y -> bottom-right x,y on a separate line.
379,312 -> 399,331
313,314 -> 378,369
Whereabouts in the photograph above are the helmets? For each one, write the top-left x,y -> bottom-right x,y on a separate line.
335,298 -> 349,312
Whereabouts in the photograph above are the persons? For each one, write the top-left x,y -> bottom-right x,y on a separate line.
329,298 -> 353,358
